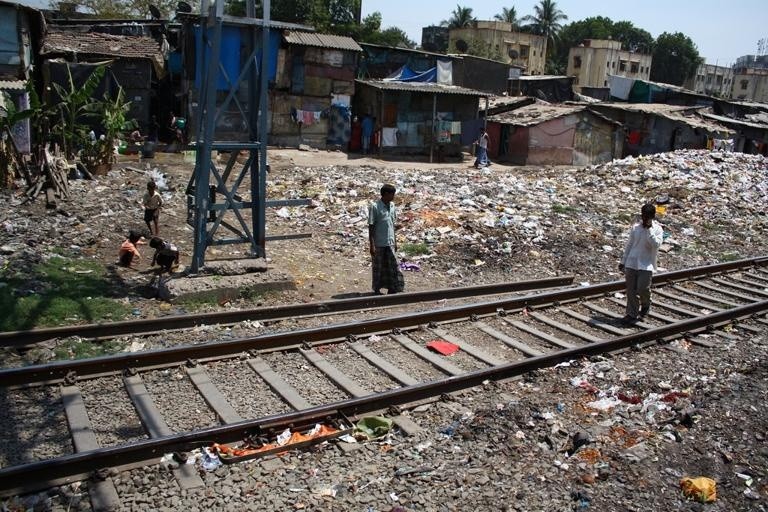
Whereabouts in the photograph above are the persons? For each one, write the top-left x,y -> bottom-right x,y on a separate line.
142,180 -> 164,236
367,184 -> 398,295
474,127 -> 491,168
118,231 -> 141,268
617,203 -> 663,325
149,237 -> 180,270
86,110 -> 186,159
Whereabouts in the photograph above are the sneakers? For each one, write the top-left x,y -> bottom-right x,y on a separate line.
641,307 -> 649,318
623,314 -> 633,322
